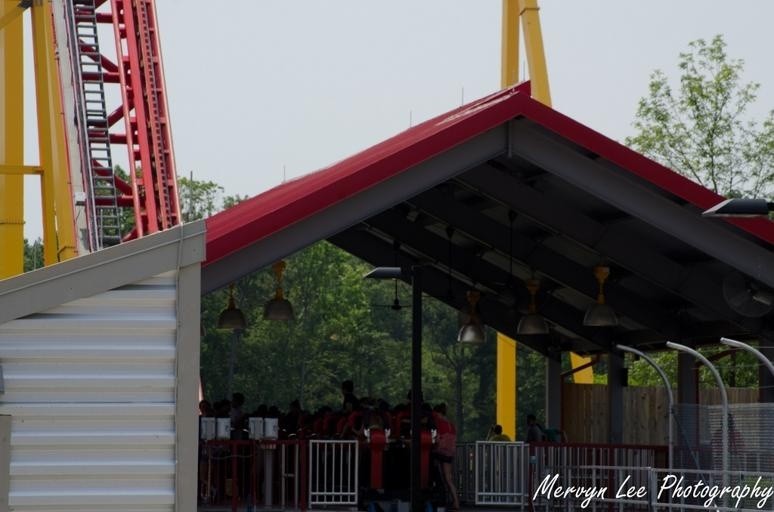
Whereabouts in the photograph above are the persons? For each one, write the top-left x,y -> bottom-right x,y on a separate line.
709,413 -> 750,472
197,376 -> 566,510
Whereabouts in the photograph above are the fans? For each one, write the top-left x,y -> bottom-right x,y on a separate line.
370,277 -> 411,310
723,270 -> 774,317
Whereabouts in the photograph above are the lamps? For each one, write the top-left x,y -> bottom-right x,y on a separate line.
457,279 -> 490,344
583,264 -> 619,326
262,258 -> 296,320
216,282 -> 246,329
516,279 -> 549,334
